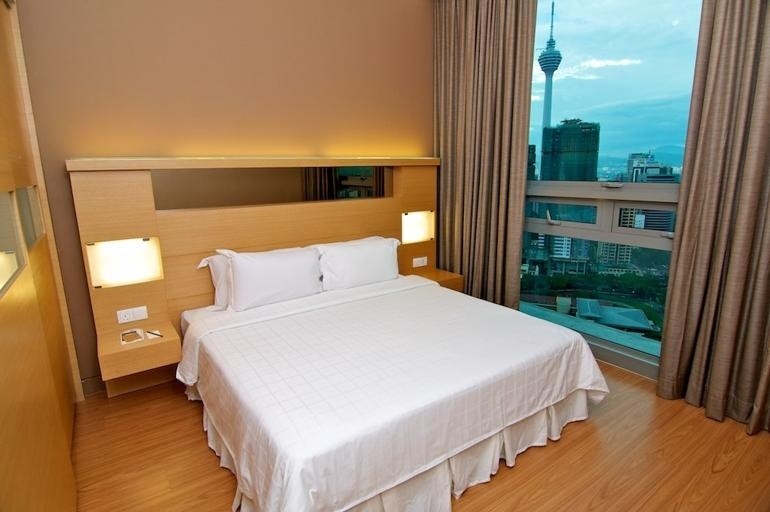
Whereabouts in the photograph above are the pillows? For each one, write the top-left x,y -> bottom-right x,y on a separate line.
196,246 -> 301,312
303,235 -> 382,249
319,236 -> 401,293
216,248 -> 324,313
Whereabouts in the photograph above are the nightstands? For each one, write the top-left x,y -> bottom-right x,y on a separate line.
421,268 -> 464,293
96,319 -> 182,399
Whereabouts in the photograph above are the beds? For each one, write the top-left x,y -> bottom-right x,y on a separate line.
180,271 -> 611,512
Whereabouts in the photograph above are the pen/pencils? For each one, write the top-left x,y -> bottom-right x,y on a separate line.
146,331 -> 164,337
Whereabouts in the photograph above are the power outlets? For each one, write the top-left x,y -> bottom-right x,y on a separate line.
117,309 -> 133,324
